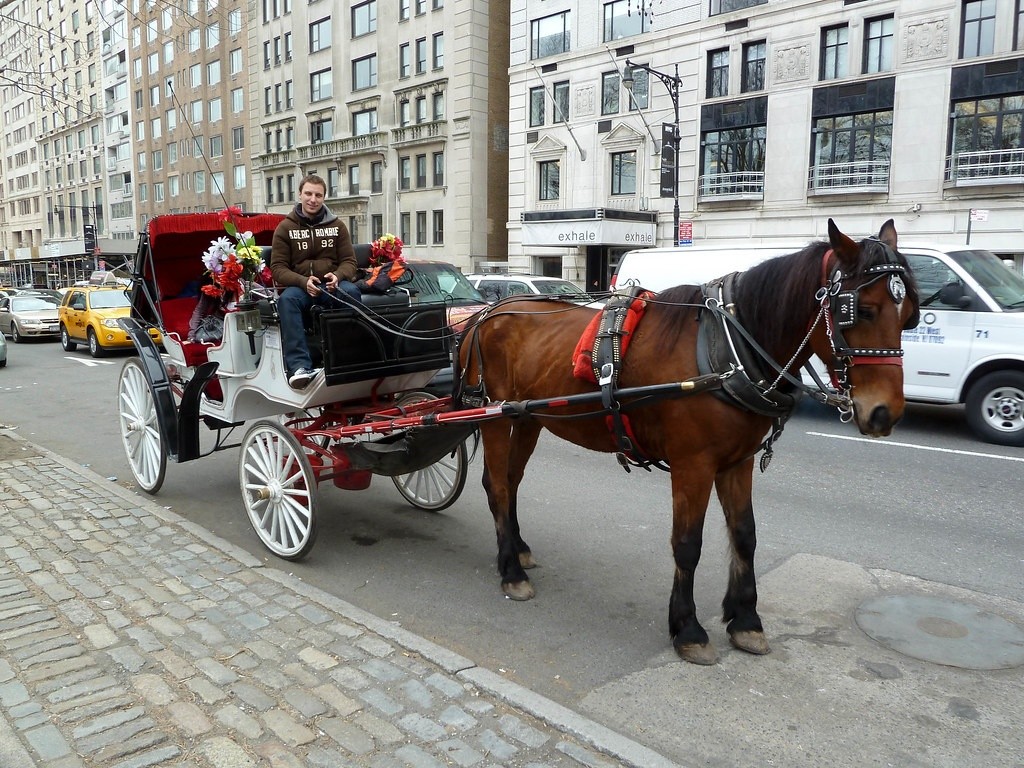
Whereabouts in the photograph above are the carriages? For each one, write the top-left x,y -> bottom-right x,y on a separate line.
118,80 -> 920,666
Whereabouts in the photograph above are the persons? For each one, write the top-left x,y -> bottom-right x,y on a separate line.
273,172 -> 363,391
926,259 -> 963,292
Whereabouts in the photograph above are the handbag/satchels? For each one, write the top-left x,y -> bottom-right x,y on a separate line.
352,260 -> 414,292
194,314 -> 224,347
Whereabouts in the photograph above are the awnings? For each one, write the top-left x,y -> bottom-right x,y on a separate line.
520,210 -> 662,279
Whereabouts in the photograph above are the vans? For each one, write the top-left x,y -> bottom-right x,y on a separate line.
607,245 -> 1024,446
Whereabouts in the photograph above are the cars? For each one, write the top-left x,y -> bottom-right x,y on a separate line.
0,271 -> 162,366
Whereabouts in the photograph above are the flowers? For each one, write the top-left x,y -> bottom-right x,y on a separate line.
370,233 -> 405,263
200,205 -> 270,301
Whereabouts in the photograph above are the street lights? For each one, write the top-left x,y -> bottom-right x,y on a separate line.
622,58 -> 682,249
54,201 -> 99,271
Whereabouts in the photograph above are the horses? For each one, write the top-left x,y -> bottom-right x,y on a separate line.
450,217 -> 920,666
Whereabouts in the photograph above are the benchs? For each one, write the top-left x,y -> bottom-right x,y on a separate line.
254,241 -> 376,294
159,295 -> 224,366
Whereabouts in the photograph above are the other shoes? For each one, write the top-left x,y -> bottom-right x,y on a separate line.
288,368 -> 318,387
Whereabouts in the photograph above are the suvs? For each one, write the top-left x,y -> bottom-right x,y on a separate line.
393,259 -> 607,349
